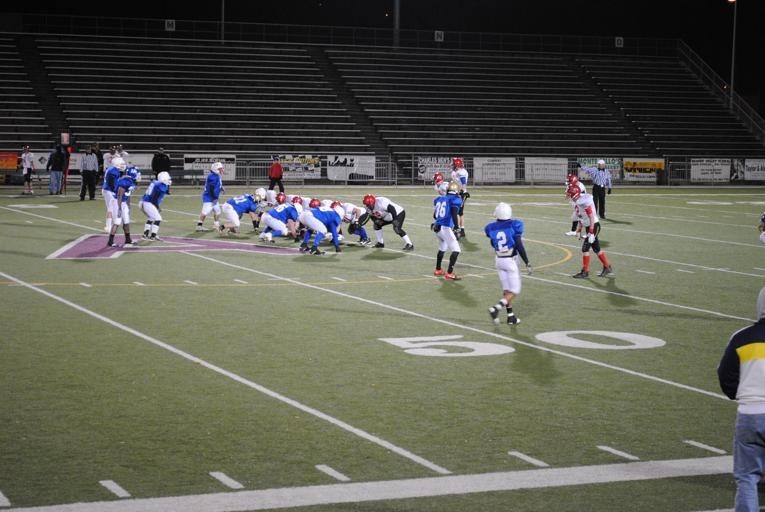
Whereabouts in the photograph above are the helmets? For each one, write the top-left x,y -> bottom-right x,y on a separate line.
158,171 -> 172,185
363,194 -> 375,209
255,194 -> 261,202
309,198 -> 321,208
255,188 -> 266,199
565,184 -> 581,200
211,162 -> 222,174
334,205 -> 344,220
434,172 -> 443,184
447,181 -> 460,194
112,158 -> 126,171
452,159 -> 462,168
495,204 -> 512,220
126,167 -> 140,179
330,201 -> 341,208
569,174 -> 578,184
276,192 -> 286,204
292,197 -> 302,204
294,203 -> 303,214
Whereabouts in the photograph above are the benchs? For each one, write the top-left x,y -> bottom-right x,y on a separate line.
576,52 -> 765,171
0,35 -> 57,153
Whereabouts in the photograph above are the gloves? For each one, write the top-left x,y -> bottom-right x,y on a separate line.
526,263 -> 532,275
608,188 -> 611,194
118,208 -> 121,218
213,199 -> 218,205
222,189 -> 225,194
577,163 -> 581,168
576,231 -> 581,240
587,232 -> 595,244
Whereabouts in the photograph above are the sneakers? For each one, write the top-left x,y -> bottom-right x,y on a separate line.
258,232 -> 265,240
507,317 -> 520,324
298,245 -> 309,251
149,237 -> 160,241
123,241 -> 138,247
403,242 -> 413,250
108,241 -> 116,247
357,239 -> 364,244
141,234 -> 149,240
565,231 -> 576,236
265,232 -> 272,241
598,266 -> 612,276
371,242 -> 384,247
488,306 -> 499,324
445,273 -> 461,280
308,248 -> 324,255
434,268 -> 446,275
362,239 -> 371,245
573,272 -> 588,278
195,225 -> 208,231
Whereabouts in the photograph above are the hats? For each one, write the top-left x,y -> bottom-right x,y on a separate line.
597,160 -> 605,164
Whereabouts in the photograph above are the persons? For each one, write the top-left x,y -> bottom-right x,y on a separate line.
757,211 -> 765,244
565,184 -> 612,279
433,172 -> 450,196
564,173 -> 589,239
195,161 -> 226,232
446,157 -> 469,239
151,146 -> 170,196
268,156 -> 286,194
430,181 -> 463,281
577,158 -> 612,219
18,145 -> 36,195
139,171 -> 172,242
716,285 -> 764,512
216,188 -> 414,256
483,201 -> 533,325
46,142 -> 142,248
